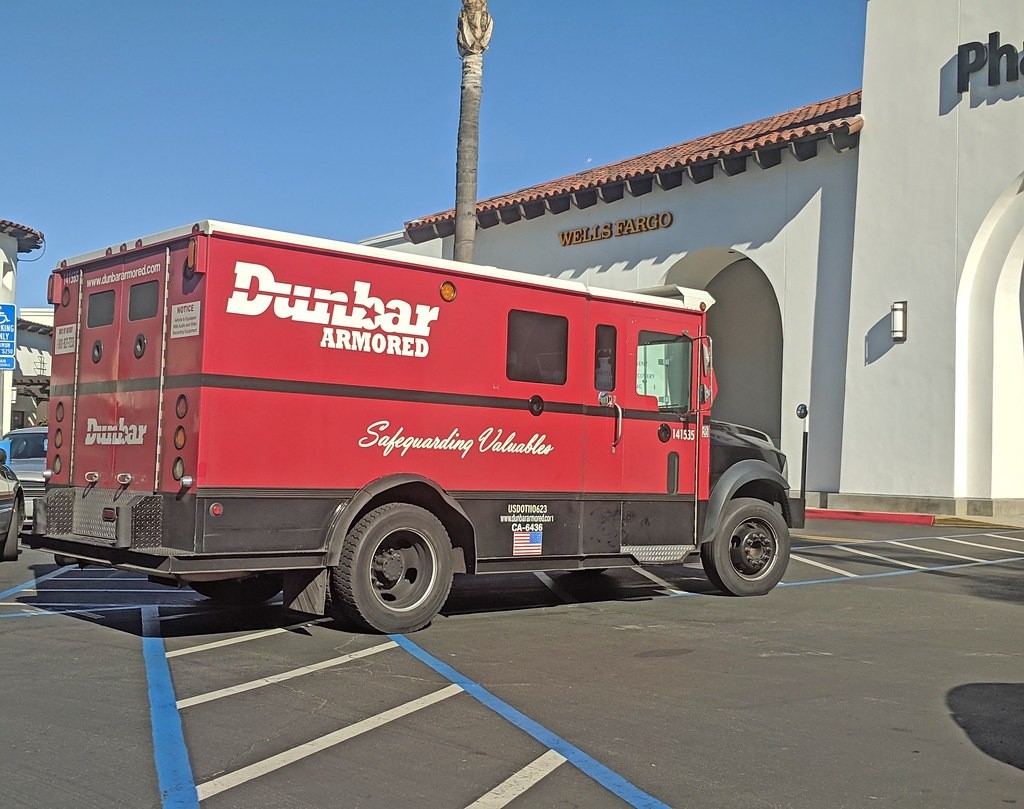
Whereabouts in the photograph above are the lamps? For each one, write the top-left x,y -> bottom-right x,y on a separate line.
11,386 -> 18,404
890,301 -> 907,342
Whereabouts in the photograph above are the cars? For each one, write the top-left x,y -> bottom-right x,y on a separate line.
3,428 -> 49,499
0,449 -> 25,562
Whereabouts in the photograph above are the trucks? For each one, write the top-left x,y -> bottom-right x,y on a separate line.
18,218 -> 809,635
